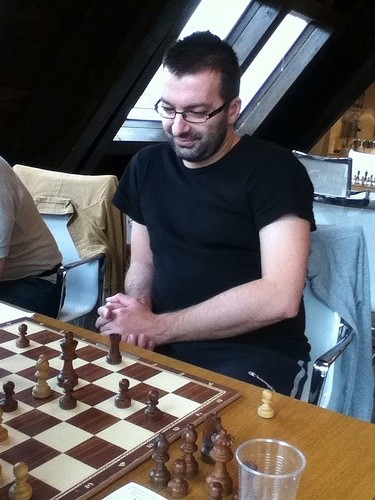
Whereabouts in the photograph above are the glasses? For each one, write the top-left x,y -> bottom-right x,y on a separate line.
154,99 -> 236,124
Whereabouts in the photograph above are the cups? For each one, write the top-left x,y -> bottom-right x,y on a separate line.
235,438 -> 307,500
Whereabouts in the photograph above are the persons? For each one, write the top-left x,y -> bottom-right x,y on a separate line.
0,157 -> 65,321
94,30 -> 318,398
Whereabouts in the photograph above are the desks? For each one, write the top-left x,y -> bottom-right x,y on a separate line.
0,299 -> 375,500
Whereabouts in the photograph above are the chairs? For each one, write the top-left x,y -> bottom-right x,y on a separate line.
300,225 -> 371,422
348,149 -> 375,185
292,150 -> 369,199
12,164 -> 126,335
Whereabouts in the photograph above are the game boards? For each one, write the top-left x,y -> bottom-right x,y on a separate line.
0,316 -> 242,500
